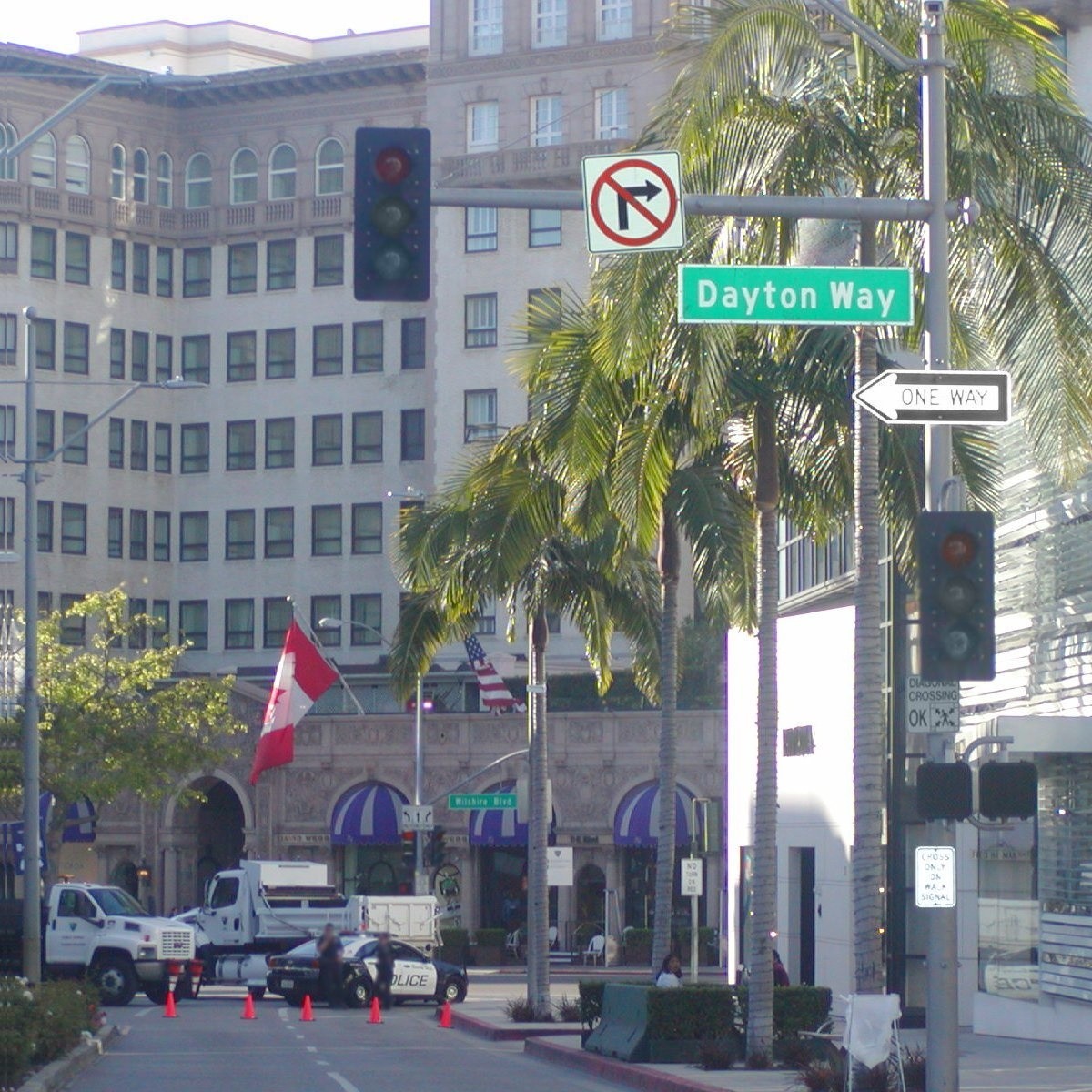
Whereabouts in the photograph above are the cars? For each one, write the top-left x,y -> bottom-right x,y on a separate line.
265,931 -> 468,1010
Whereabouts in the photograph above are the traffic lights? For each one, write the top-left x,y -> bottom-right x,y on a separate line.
914,508 -> 999,683
978,759 -> 1038,821
917,762 -> 973,823
353,124 -> 434,305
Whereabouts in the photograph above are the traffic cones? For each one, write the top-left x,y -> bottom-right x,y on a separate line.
299,995 -> 316,1021
238,995 -> 257,1020
365,997 -> 384,1023
436,1001 -> 454,1029
166,962 -> 182,995
189,960 -> 208,998
162,992 -> 181,1019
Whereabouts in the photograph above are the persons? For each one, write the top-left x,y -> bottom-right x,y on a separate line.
772,949 -> 791,987
358,931 -> 397,1009
316,922 -> 349,1009
170,905 -> 192,918
656,954 -> 682,989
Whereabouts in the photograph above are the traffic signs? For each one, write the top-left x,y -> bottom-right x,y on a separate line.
854,370 -> 1012,427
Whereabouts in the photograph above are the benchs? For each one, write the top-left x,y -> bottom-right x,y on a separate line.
581,933 -> 606,970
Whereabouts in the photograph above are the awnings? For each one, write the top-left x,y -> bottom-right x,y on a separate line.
40,790 -> 97,842
470,778 -> 556,845
613,776 -> 701,848
333,782 -> 412,848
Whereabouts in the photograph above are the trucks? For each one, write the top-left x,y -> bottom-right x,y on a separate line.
0,817 -> 199,1007
167,860 -> 444,1002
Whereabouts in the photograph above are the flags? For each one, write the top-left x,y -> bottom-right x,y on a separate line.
251,615 -> 338,786
462,630 -> 528,718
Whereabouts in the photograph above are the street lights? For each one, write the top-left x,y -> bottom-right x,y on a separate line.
318,617 -> 427,899
20,304 -> 211,989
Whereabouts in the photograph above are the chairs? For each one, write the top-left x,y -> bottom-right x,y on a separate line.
548,926 -> 558,948
792,991 -> 907,1092
504,929 -> 521,961
